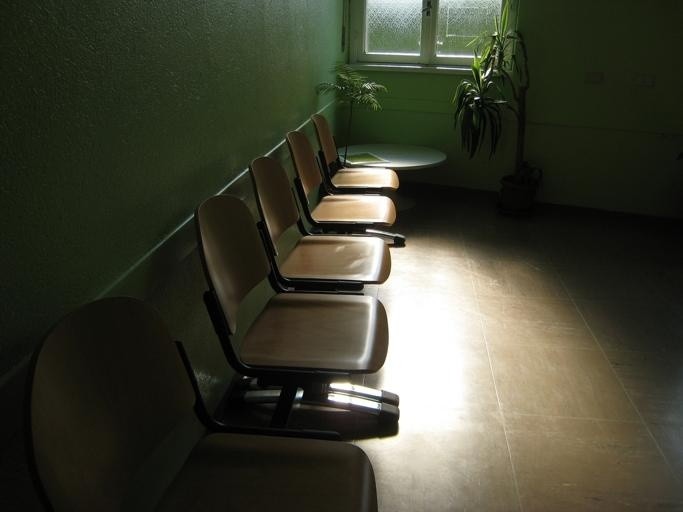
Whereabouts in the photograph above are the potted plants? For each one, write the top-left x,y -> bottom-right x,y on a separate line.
451,0 -> 542,216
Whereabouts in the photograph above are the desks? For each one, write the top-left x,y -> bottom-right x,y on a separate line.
337,144 -> 447,213
